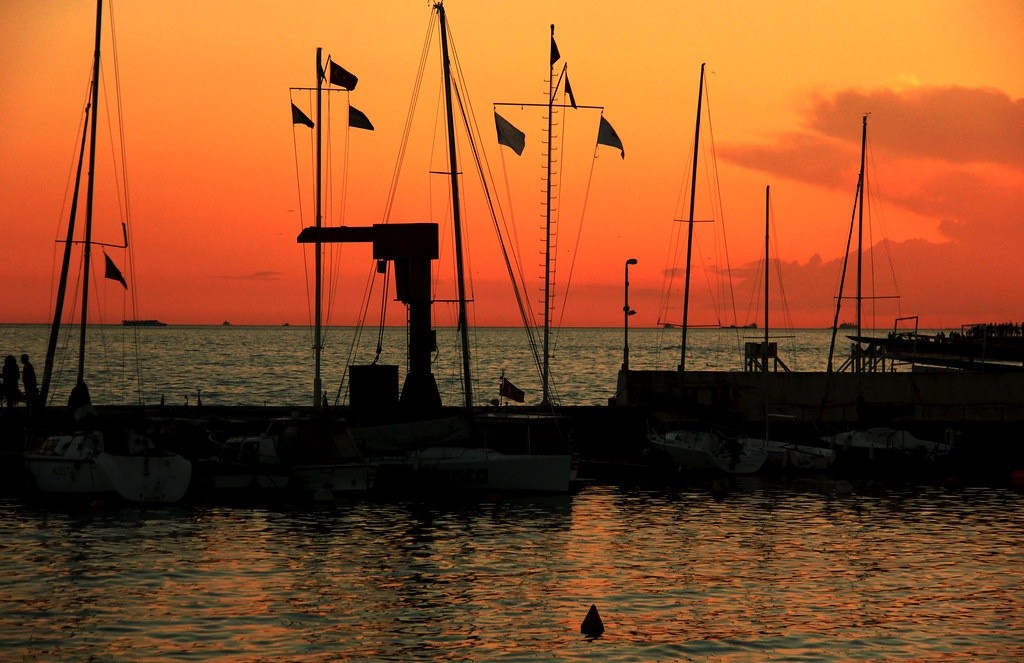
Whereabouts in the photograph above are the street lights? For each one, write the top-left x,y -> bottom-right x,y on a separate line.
622,257 -> 639,373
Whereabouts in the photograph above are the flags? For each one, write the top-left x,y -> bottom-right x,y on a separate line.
552,38 -> 560,65
105,255 -> 128,289
598,116 -> 625,159
330,60 -> 358,90
502,378 -> 525,403
495,112 -> 525,156
349,105 -> 375,130
564,74 -> 576,109
292,103 -> 315,129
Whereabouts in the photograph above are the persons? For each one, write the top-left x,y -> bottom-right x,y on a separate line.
1,355 -> 20,408
889,322 -> 1024,343
22,355 -> 37,398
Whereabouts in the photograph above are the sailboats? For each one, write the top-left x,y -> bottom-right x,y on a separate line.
0,1 -> 1023,508
